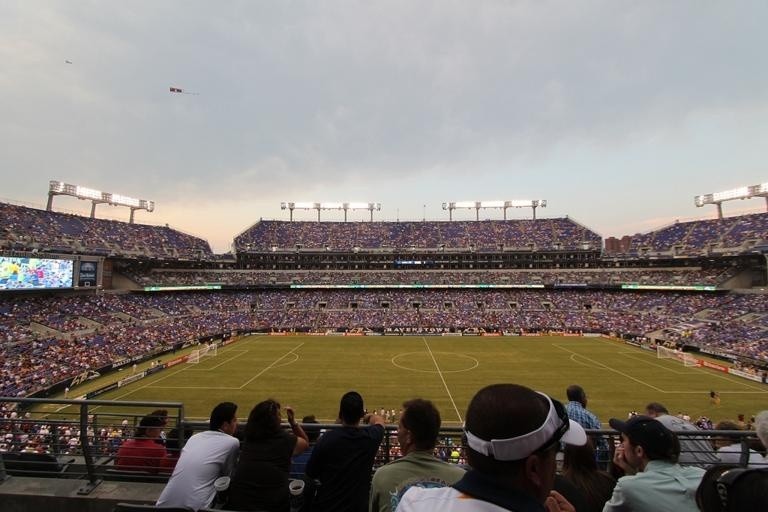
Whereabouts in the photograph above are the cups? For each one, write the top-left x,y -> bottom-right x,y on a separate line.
213,476 -> 231,503
289,479 -> 305,508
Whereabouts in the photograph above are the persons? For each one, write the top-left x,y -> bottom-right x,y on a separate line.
1,370 -> 768,511
221,209 -> 606,332
0,202 -> 221,385
606,218 -> 768,375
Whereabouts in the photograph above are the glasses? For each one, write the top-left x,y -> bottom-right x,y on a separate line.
530,397 -> 570,455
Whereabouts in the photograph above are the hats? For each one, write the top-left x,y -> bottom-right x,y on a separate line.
609,413 -> 680,462
460,390 -> 593,460
